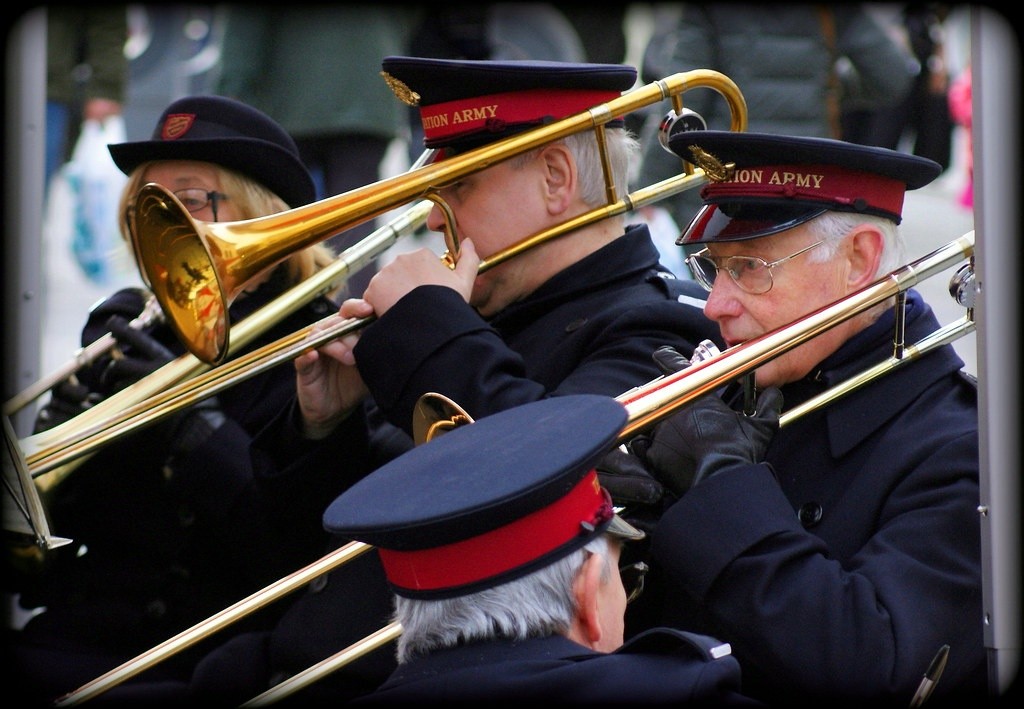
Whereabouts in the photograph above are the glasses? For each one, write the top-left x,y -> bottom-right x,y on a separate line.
126,187 -> 234,218
685,226 -> 850,295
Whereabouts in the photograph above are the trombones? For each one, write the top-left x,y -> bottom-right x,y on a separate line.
0,69 -> 749,548
1,296 -> 189,491
50,229 -> 979,709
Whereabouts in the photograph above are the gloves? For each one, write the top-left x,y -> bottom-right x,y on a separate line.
596,449 -> 677,546
628,346 -> 784,496
30,380 -> 105,437
100,315 -> 220,439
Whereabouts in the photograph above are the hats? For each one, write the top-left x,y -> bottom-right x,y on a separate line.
669,130 -> 942,244
323,393 -> 646,600
107,96 -> 317,209
379,56 -> 638,171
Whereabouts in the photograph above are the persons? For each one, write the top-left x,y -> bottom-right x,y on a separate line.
586,127 -> 988,699
0,96 -> 346,709
193,53 -> 728,709
1,1 -> 978,398
315,397 -> 743,709
